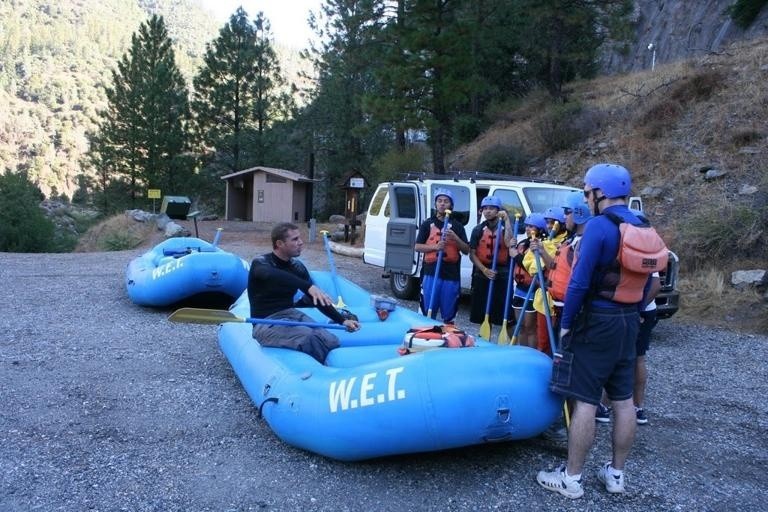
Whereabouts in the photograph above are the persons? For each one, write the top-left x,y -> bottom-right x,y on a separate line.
469,196 -> 516,342
247,221 -> 361,365
509,191 -> 661,426
414,189 -> 469,326
535,164 -> 652,500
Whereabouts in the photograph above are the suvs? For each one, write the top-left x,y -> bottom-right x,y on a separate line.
362,172 -> 680,331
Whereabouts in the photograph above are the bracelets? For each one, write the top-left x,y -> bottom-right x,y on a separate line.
482,268 -> 487,274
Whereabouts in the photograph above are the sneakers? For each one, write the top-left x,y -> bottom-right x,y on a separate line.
537,465 -> 584,500
635,407 -> 649,424
597,461 -> 625,494
596,404 -> 610,421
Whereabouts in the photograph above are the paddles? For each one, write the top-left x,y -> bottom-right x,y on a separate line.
477,213 -> 560,346
167,308 -> 350,330
529,229 -> 572,434
320,229 -> 347,308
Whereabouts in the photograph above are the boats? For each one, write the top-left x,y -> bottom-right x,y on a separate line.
216,268 -> 566,462
125,236 -> 251,309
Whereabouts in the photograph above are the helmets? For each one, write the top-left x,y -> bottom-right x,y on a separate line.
480,196 -> 502,209
434,187 -> 454,205
564,191 -> 590,225
582,163 -> 632,200
524,212 -> 546,229
545,206 -> 565,223
